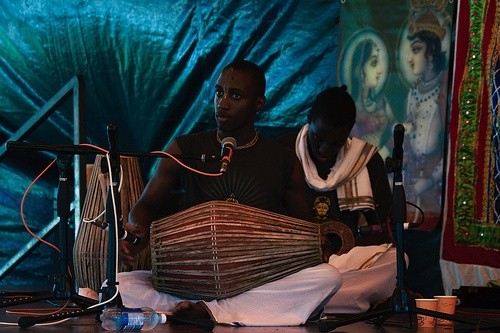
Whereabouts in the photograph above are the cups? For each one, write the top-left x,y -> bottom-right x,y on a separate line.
415,298 -> 439,328
434,295 -> 457,325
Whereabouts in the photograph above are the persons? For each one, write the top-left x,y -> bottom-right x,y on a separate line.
272,84 -> 416,315
100,60 -> 341,327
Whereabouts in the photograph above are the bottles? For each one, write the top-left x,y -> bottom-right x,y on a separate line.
100,306 -> 167,331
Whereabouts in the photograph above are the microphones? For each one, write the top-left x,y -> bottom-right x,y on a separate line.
220,137 -> 237,175
389,148 -> 408,223
88,218 -> 141,245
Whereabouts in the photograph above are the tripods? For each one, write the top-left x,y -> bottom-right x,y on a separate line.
317,125 -> 487,333
0,123 -> 220,333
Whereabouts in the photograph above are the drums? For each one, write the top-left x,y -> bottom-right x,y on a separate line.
150,200 -> 325,301
72,154 -> 147,302
